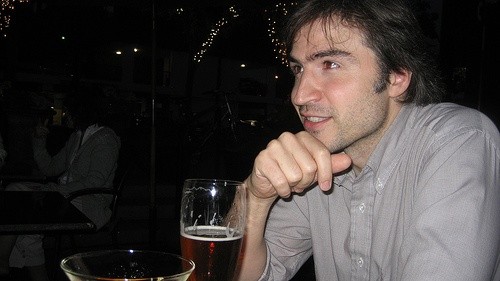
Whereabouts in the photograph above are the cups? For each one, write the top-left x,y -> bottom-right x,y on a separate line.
60,249 -> 196,281
180,179 -> 247,281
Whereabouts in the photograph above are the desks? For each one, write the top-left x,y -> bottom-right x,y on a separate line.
0,191 -> 97,235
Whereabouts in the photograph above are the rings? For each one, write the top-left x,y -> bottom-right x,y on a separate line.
39,125 -> 41,129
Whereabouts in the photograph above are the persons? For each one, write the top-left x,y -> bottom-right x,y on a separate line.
0,84 -> 119,281
220,1 -> 500,281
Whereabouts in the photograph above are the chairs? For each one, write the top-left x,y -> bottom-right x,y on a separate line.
50,181 -> 122,281
